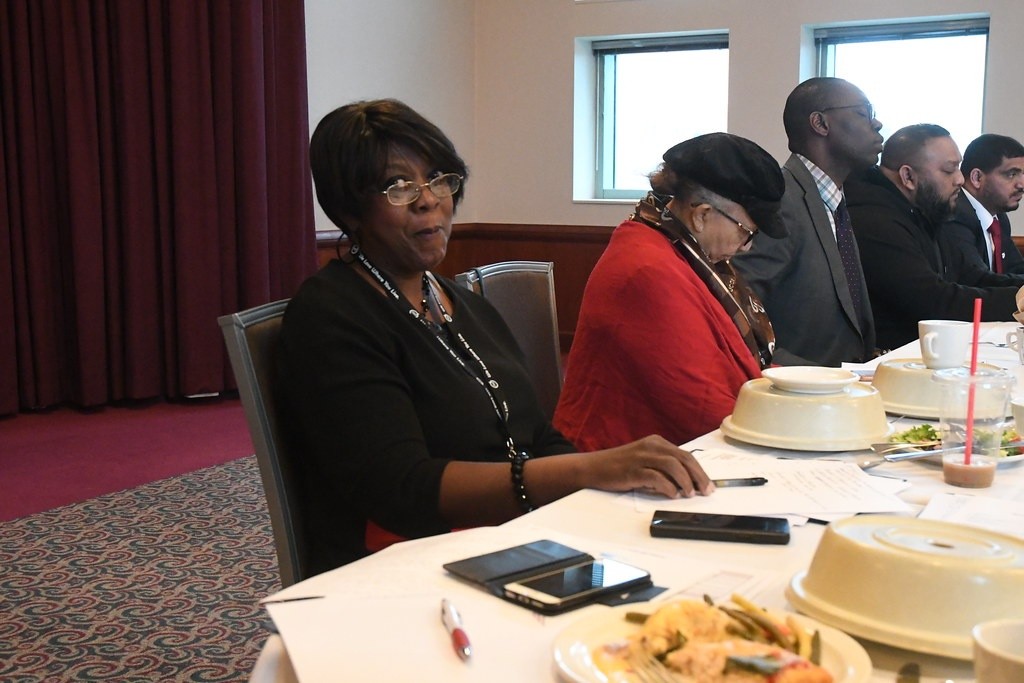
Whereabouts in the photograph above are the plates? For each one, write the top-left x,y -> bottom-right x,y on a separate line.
898,442 -> 1024,463
553,613 -> 875,683
761,366 -> 860,394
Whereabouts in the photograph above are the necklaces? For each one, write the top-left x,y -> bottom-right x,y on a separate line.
419,274 -> 430,317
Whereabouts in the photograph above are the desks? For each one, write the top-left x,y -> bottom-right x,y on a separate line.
249,322 -> 1024,683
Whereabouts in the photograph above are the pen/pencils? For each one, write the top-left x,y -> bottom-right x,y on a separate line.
678,477 -> 768,488
441,598 -> 472,662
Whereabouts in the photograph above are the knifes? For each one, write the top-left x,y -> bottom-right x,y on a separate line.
884,442 -> 1024,461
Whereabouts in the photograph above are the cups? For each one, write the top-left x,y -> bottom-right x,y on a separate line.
931,369 -> 1016,490
1007,328 -> 1024,365
918,320 -> 973,370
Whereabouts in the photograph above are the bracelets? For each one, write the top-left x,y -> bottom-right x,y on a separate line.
510,452 -> 534,512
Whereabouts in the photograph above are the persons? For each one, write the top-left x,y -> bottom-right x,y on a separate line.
271,96 -> 716,575
844,125 -> 1024,352
551,134 -> 786,452
729,76 -> 884,371
942,133 -> 1024,289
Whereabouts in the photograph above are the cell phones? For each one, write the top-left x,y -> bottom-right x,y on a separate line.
650,511 -> 790,546
502,558 -> 651,612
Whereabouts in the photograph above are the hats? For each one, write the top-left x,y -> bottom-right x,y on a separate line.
663,132 -> 793,237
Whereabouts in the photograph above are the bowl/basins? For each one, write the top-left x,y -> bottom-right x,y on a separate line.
973,619 -> 1024,683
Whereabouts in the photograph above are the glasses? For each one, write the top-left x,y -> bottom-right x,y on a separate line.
691,201 -> 760,247
808,103 -> 874,124
377,172 -> 465,206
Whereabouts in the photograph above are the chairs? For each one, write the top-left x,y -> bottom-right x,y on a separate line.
454,261 -> 563,422
215,298 -> 308,589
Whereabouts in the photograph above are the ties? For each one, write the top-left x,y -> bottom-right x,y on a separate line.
833,195 -> 865,337
989,216 -> 1003,275
